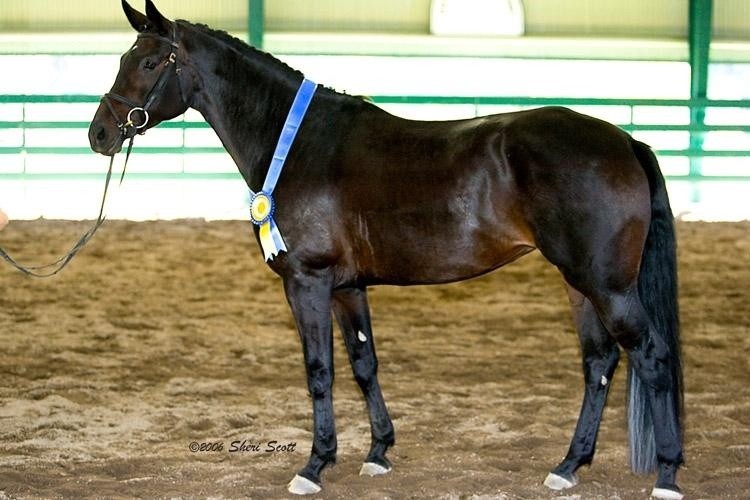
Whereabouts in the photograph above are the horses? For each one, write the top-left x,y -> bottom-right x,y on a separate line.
87,0 -> 686,500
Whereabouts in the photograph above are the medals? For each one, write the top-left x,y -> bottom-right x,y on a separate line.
249,191 -> 275,225
